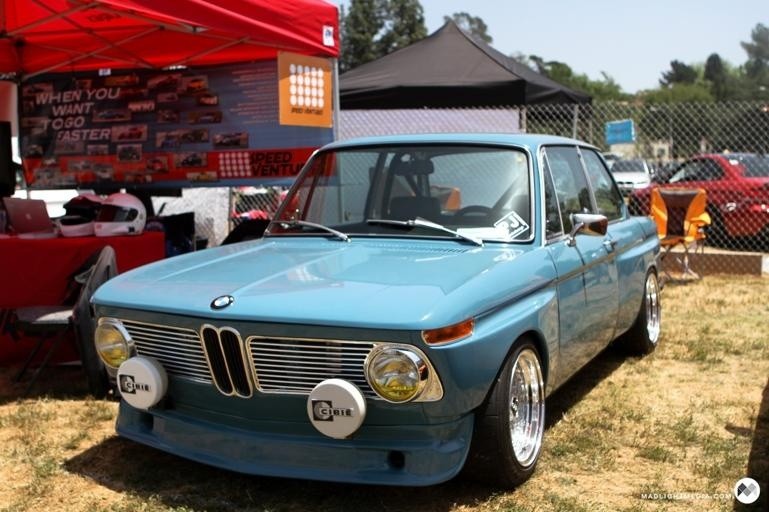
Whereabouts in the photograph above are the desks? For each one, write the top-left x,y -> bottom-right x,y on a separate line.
0,231 -> 167,367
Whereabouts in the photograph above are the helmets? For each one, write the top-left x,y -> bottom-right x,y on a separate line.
59,194 -> 104,238
95,193 -> 147,237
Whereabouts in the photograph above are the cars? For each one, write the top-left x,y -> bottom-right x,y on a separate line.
603,152 -> 769,251
90,132 -> 661,486
28,73 -> 242,179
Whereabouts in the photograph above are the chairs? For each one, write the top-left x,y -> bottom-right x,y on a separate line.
650,187 -> 711,285
385,194 -> 440,227
14,246 -> 114,399
